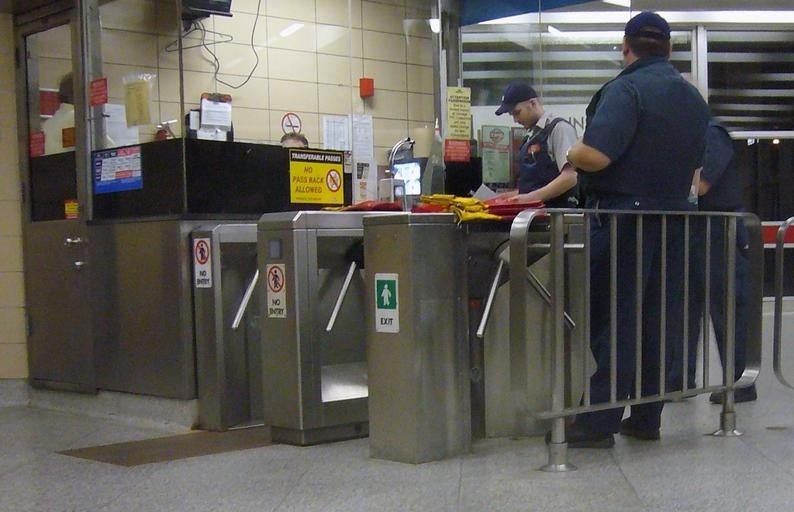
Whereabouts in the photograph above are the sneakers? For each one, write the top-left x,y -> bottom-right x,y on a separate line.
619,416 -> 661,440
710,384 -> 756,403
665,385 -> 697,402
545,422 -> 616,449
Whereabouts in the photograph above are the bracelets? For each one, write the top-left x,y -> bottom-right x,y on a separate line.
566,145 -> 570,161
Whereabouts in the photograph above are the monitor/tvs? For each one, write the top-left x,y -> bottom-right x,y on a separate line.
392,158 -> 428,196
183,0 -> 233,17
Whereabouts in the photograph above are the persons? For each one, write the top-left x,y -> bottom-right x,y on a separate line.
279,132 -> 308,147
494,83 -> 583,208
39,71 -> 114,154
664,118 -> 758,403
544,18 -> 708,450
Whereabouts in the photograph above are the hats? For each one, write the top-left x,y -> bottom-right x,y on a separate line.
625,12 -> 672,40
495,83 -> 538,116
